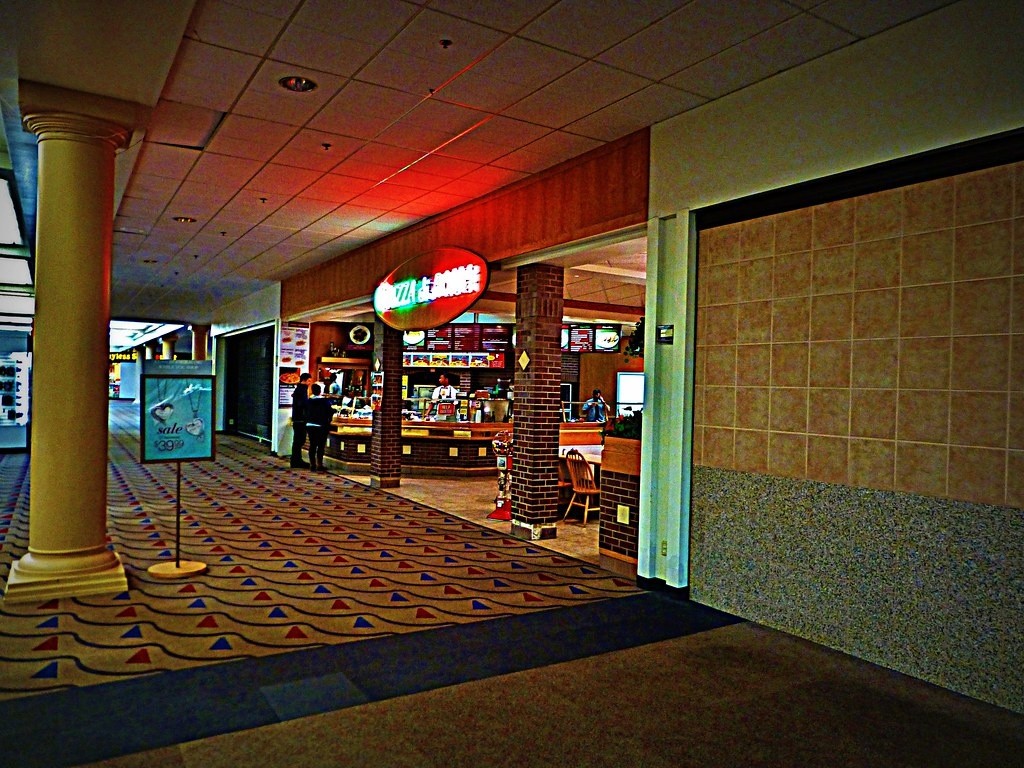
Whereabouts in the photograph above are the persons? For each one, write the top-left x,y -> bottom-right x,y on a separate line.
329,373 -> 342,398
290,373 -> 334,471
582,388 -> 610,421
425,372 -> 459,418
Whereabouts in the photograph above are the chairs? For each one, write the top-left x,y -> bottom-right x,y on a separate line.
558,449 -> 600,528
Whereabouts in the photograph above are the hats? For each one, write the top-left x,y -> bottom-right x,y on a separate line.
593,390 -> 600,394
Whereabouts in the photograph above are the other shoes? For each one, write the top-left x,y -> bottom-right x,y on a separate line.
318,466 -> 327,471
290,458 -> 310,469
311,466 -> 316,471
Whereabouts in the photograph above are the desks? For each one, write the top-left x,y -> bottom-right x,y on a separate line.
558,454 -> 602,522
